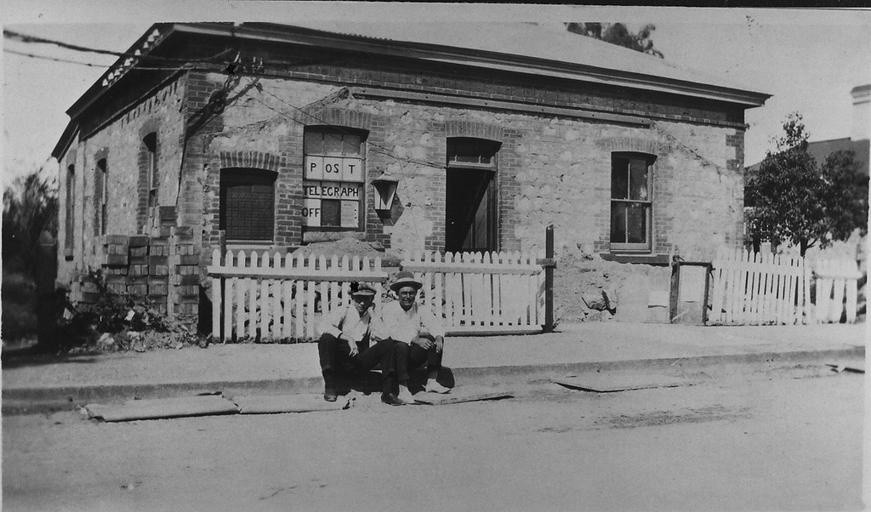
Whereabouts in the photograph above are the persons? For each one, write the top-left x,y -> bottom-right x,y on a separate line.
318,272 -> 450,406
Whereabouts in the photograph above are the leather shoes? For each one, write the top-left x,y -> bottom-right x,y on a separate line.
426,380 -> 450,394
381,390 -> 414,406
324,383 -> 338,402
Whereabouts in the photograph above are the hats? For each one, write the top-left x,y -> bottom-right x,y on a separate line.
389,272 -> 423,290
347,281 -> 377,296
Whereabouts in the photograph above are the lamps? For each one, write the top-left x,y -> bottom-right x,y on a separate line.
372,171 -> 400,218
